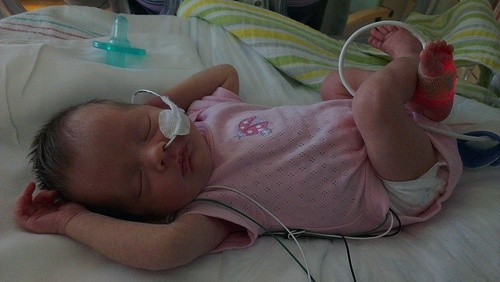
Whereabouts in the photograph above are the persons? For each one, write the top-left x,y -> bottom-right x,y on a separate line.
13,23 -> 465,273
